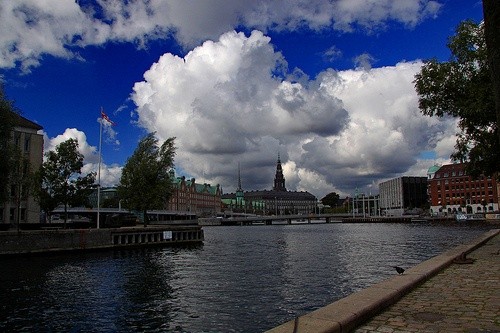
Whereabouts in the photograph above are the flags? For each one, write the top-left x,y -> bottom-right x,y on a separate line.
102,112 -> 116,126
